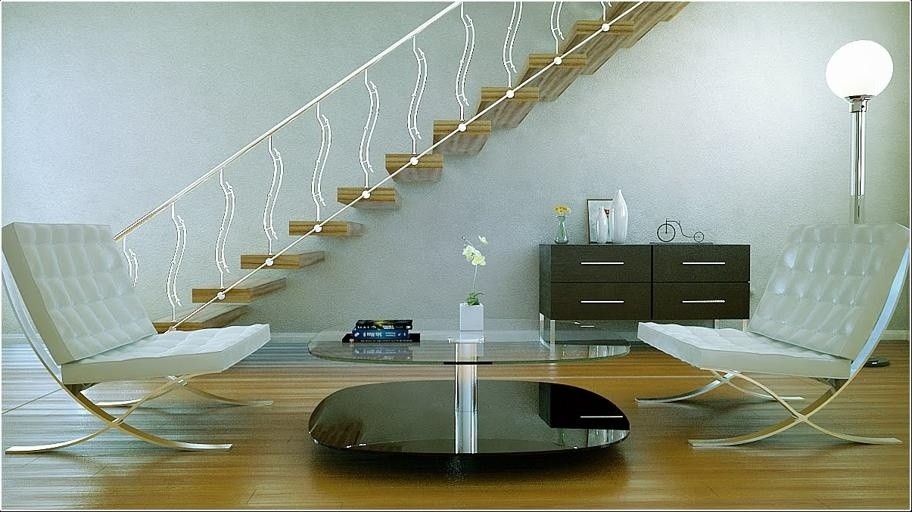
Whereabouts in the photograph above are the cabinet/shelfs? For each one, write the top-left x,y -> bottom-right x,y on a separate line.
652,243 -> 749,345
537,244 -> 652,346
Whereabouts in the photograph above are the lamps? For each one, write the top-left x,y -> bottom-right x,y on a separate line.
825,40 -> 893,368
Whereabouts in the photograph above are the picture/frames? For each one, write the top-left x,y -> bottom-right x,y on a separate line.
587,198 -> 613,242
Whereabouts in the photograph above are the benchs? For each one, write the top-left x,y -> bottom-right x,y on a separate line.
4,221 -> 273,455
634,221 -> 910,446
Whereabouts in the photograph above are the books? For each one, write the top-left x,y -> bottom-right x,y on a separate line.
342,319 -> 421,344
353,343 -> 414,360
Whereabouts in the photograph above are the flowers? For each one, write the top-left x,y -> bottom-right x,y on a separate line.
553,206 -> 568,215
460,235 -> 487,304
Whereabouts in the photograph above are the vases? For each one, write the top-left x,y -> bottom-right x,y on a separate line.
555,216 -> 568,244
609,189 -> 627,242
596,207 -> 608,244
459,302 -> 484,331
